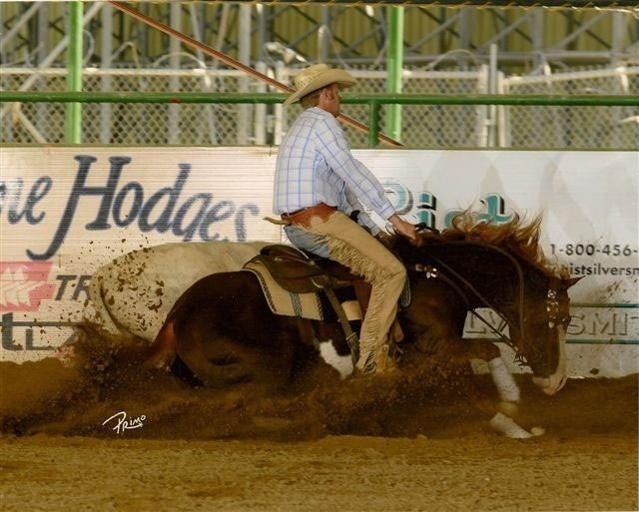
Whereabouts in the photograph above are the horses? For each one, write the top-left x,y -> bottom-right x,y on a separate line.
0,197 -> 587,436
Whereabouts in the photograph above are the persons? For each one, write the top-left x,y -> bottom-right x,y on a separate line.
272,63 -> 418,378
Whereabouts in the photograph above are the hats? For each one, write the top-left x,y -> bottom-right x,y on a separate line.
281,63 -> 358,107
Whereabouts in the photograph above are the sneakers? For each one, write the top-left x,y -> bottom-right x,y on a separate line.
352,350 -> 396,377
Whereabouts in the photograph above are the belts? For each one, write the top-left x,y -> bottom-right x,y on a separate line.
281,207 -> 310,217
